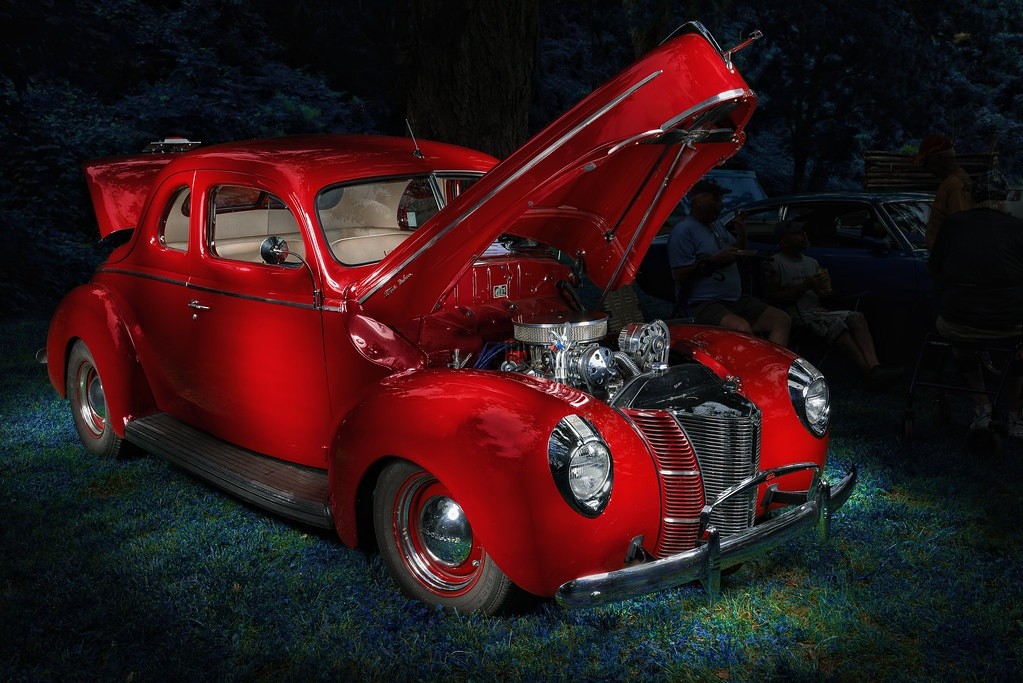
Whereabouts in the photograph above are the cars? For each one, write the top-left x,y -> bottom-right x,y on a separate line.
571,190 -> 938,370
31,18 -> 860,620
645,167 -> 782,237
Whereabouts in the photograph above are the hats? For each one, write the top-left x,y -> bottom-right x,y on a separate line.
913,135 -> 952,165
773,221 -> 808,243
973,169 -> 1007,201
690,178 -> 732,197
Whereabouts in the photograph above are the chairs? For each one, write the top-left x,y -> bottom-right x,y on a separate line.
668,247 -> 875,375
329,234 -> 413,264
216,240 -> 303,266
860,218 -> 888,242
907,269 -> 1023,423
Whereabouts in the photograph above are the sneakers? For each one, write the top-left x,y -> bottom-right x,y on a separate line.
969,405 -> 994,430
1008,410 -> 1023,438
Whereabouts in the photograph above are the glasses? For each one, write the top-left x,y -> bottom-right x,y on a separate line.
714,231 -> 724,250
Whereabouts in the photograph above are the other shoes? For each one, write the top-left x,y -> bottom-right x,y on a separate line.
863,368 -> 903,389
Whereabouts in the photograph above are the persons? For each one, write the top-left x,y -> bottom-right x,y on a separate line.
921,135 -> 1023,437
764,222 -> 906,393
668,180 -> 790,348
